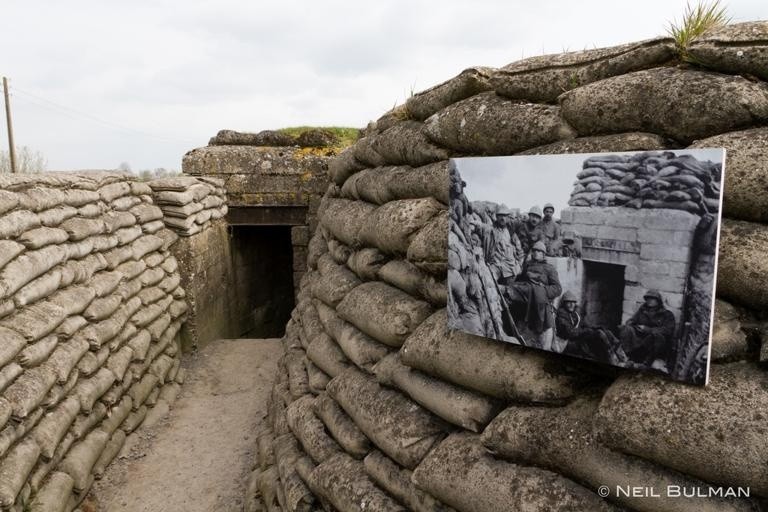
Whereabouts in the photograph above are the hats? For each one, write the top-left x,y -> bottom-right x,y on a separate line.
561,291 -> 578,303
496,201 -> 555,227
532,241 -> 546,252
643,287 -> 662,300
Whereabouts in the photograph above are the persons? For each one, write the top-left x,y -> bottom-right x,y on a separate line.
488,201 -> 675,374
